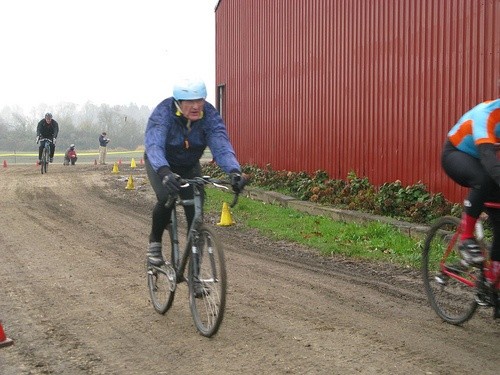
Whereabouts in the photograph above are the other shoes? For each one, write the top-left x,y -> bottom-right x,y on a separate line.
39,160 -> 41,165
50,158 -> 52,162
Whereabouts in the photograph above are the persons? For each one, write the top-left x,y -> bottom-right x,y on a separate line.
99,132 -> 110,164
440,98 -> 500,308
63,144 -> 77,165
37,113 -> 59,165
143,76 -> 242,298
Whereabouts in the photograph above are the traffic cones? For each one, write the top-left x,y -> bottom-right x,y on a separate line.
118,159 -> 144,167
110,161 -> 120,174
216,200 -> 236,226
35,160 -> 39,167
94,159 -> 98,165
0,322 -> 14,348
3,160 -> 8,168
130,158 -> 137,167
125,173 -> 135,190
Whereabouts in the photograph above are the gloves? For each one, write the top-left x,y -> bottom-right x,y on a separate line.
162,174 -> 183,195
229,173 -> 244,194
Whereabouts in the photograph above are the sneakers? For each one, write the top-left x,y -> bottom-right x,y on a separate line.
194,280 -> 209,296
147,238 -> 164,267
459,239 -> 487,264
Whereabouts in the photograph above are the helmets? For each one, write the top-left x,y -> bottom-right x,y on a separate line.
173,78 -> 206,101
70,144 -> 75,149
45,113 -> 52,119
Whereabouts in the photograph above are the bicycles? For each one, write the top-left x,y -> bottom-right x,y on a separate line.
146,167 -> 254,338
422,200 -> 500,326
